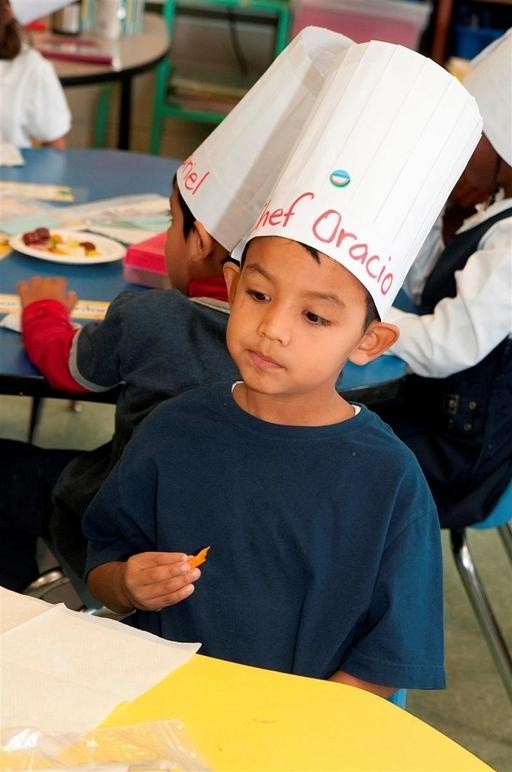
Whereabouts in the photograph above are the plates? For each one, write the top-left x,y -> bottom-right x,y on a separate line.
9,229 -> 126,266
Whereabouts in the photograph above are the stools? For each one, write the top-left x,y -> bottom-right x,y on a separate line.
148,0 -> 289,156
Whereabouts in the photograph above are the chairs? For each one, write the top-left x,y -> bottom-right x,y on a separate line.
450,485 -> 512,704
389,688 -> 407,708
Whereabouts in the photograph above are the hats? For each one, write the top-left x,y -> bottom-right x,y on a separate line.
242,40 -> 482,321
464,29 -> 512,169
178,25 -> 358,260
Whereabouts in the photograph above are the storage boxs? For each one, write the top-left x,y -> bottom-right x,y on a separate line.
291,0 -> 433,53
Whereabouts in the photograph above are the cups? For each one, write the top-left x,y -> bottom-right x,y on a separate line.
95,0 -> 122,38
50,1 -> 82,36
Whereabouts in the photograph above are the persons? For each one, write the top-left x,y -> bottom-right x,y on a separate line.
0,169 -> 242,600
0,0 -> 75,151
332,131 -> 511,529
82,234 -> 448,701
